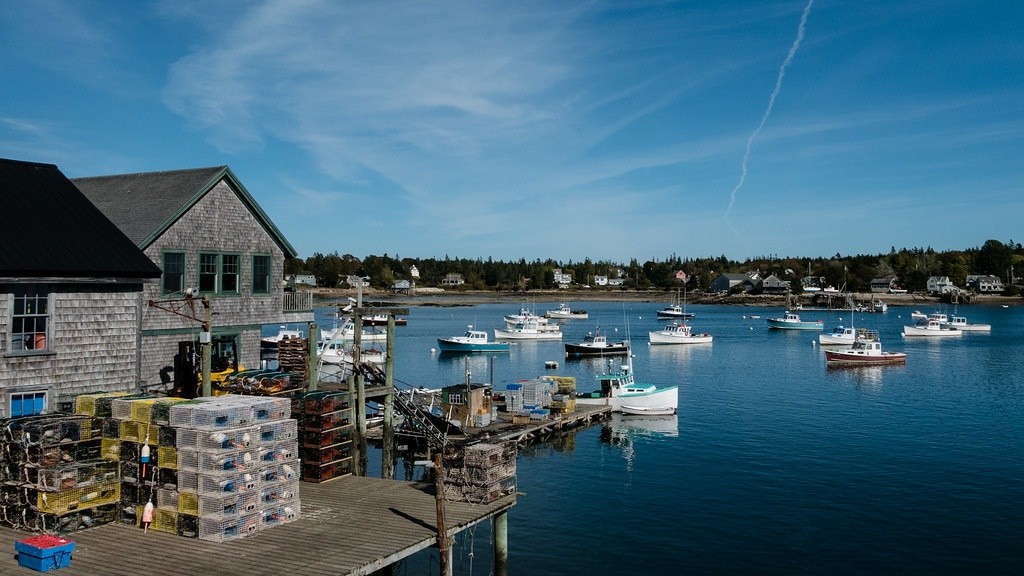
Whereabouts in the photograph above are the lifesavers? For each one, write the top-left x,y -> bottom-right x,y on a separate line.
337,350 -> 343,356
685,332 -> 688,336
681,324 -> 685,329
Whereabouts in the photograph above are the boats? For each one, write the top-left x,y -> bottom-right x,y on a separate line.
320,315 -> 388,343
317,339 -> 388,365
436,313 -> 511,352
611,415 -> 679,439
564,312 -> 630,357
503,290 -> 560,331
656,288 -> 697,320
824,337 -> 908,364
494,293 -> 563,340
942,288 -> 978,305
766,309 -> 824,330
648,286 -> 713,345
341,295 -> 408,326
261,323 -> 308,350
1001,304 -> 1009,308
904,317 -> 963,336
911,310 -> 927,318
853,293 -> 888,313
544,298 -> 589,319
574,304 -> 680,415
818,306 -> 880,345
913,313 -> 991,331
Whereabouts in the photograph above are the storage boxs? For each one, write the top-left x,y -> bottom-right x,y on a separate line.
505,376 -> 576,421
474,409 -> 490,427
513,412 -> 530,423
15,535 -> 74,573
